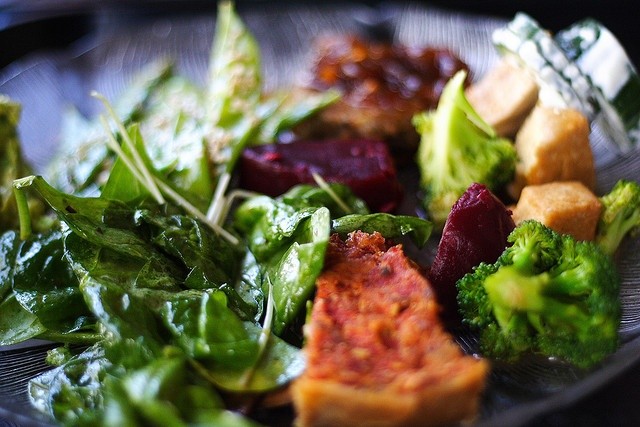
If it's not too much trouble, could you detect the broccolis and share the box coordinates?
[456,219,624,372]
[410,70,519,225]
